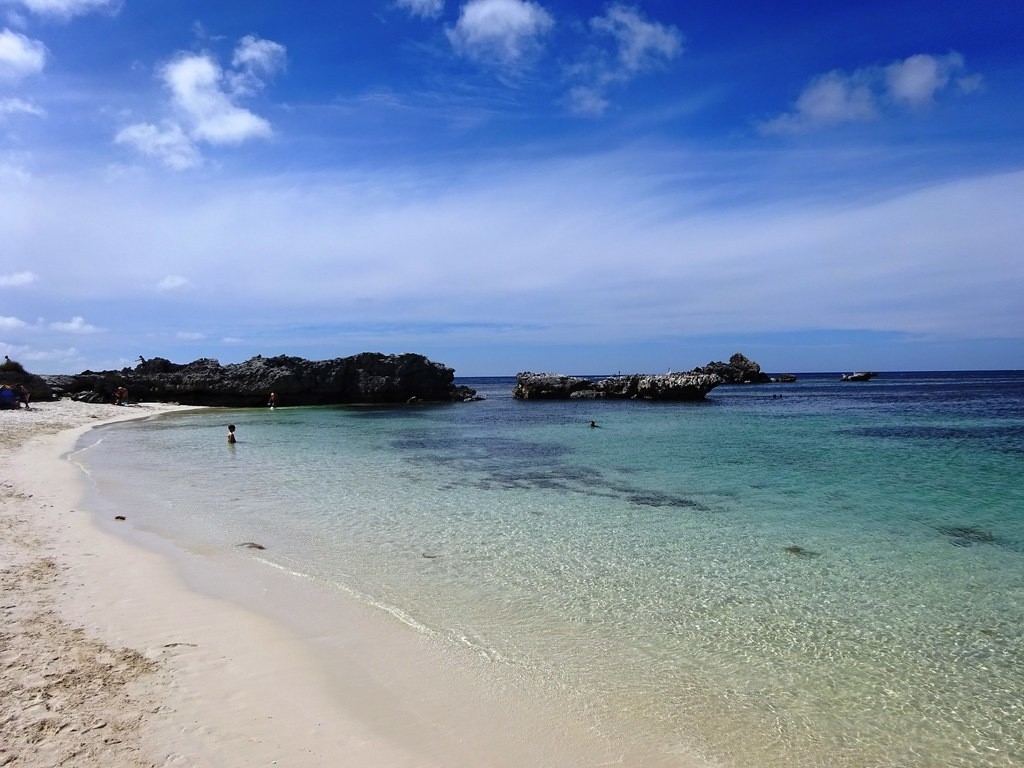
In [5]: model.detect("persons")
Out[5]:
[227,425,236,444]
[5,356,10,362]
[589,420,596,427]
[135,355,145,364]
[268,391,276,408]
[11,383,30,409]
[115,386,129,404]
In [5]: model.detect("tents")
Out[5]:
[0,384,16,410]
